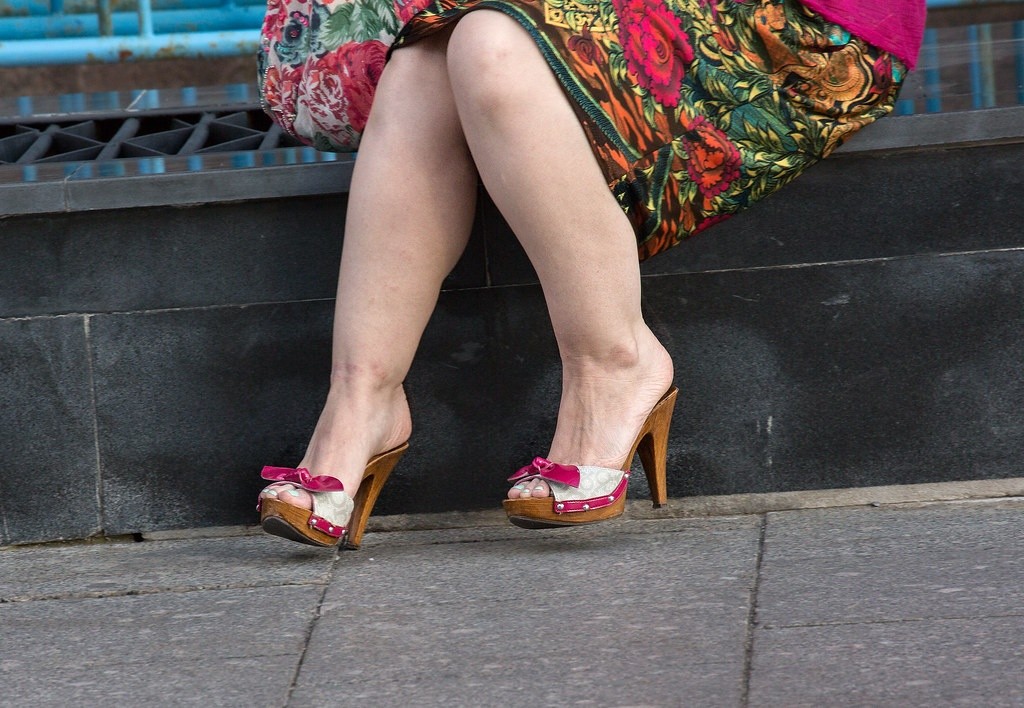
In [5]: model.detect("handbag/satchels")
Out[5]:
[257,0,434,153]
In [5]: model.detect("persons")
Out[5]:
[254,0,929,550]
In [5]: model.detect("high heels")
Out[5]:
[256,441,409,550]
[502,386,679,529]
